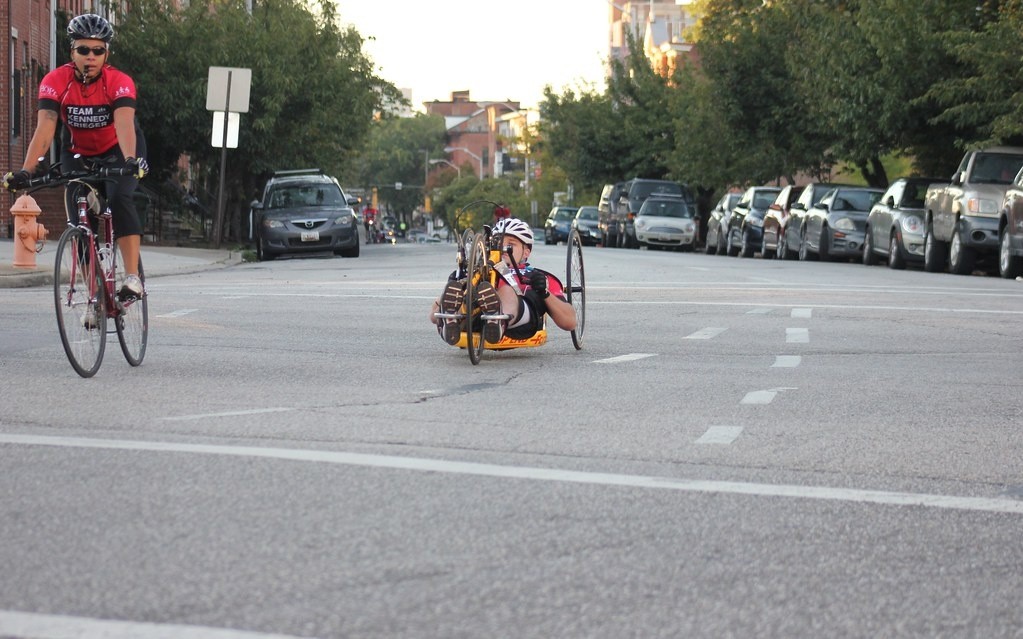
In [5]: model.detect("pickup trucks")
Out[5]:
[923,146,1023,274]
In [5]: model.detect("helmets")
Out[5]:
[67,14,113,43]
[492,218,535,244]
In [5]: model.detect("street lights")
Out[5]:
[470,101,531,196]
[443,144,484,182]
[429,157,461,180]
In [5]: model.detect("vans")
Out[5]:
[598,178,694,249]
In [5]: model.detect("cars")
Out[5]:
[799,187,886,261]
[864,177,952,269]
[543,206,602,245]
[631,194,702,252]
[378,215,441,243]
[250,168,361,260]
[999,164,1023,279]
[705,183,864,260]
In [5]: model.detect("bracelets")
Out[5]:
[435,301,441,306]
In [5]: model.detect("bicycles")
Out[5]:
[12,153,150,378]
[364,218,377,244]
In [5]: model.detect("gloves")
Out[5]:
[3,172,28,193]
[448,267,467,286]
[131,157,149,181]
[530,270,550,300]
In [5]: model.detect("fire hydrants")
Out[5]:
[10,191,50,267]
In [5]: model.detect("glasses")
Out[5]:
[73,45,107,56]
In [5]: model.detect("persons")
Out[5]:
[430,219,577,346]
[4,13,149,331]
[492,202,511,223]
[362,203,378,244]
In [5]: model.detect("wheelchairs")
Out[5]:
[433,200,587,365]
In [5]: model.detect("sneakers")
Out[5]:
[476,282,503,344]
[120,275,143,300]
[80,309,97,329]
[442,280,464,345]
